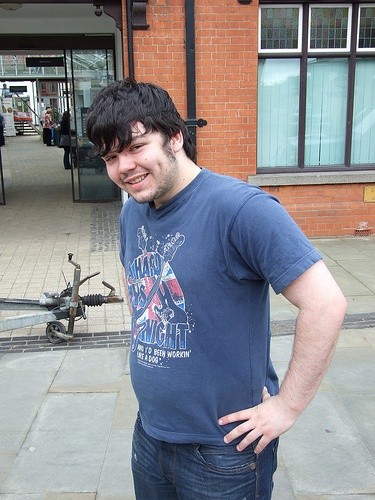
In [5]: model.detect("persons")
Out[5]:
[39,105,53,147]
[58,110,74,170]
[83,79,348,500]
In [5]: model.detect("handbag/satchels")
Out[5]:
[60,135,70,147]
